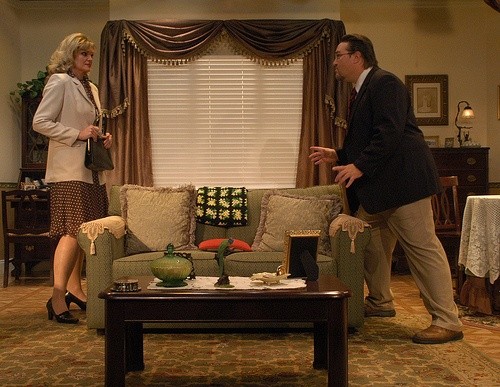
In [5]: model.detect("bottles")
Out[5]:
[150,243,192,287]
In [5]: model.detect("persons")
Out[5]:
[308,33,463,344]
[32,32,113,324]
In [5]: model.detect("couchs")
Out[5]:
[77,184,371,332]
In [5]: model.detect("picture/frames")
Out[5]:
[424,136,439,148]
[445,138,454,148]
[405,74,449,126]
[282,229,322,278]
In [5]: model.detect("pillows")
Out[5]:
[120,184,198,256]
[199,239,252,252]
[251,190,343,254]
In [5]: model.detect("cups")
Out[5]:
[445,137,454,147]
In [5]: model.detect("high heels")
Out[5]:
[64,290,86,312]
[47,297,79,324]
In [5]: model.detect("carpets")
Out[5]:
[0,312,500,387]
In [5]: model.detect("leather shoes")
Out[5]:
[364,301,396,317]
[413,324,463,344]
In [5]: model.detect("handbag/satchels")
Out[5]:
[85,112,114,171]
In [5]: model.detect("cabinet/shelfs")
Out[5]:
[10,72,51,276]
[430,148,490,274]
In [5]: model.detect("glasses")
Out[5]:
[334,53,357,60]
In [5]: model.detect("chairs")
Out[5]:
[432,177,464,294]
[1,190,50,287]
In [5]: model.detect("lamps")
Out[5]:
[455,101,474,147]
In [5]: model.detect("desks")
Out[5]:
[458,195,500,314]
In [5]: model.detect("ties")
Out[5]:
[349,88,357,124]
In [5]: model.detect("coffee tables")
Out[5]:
[98,276,353,386]
